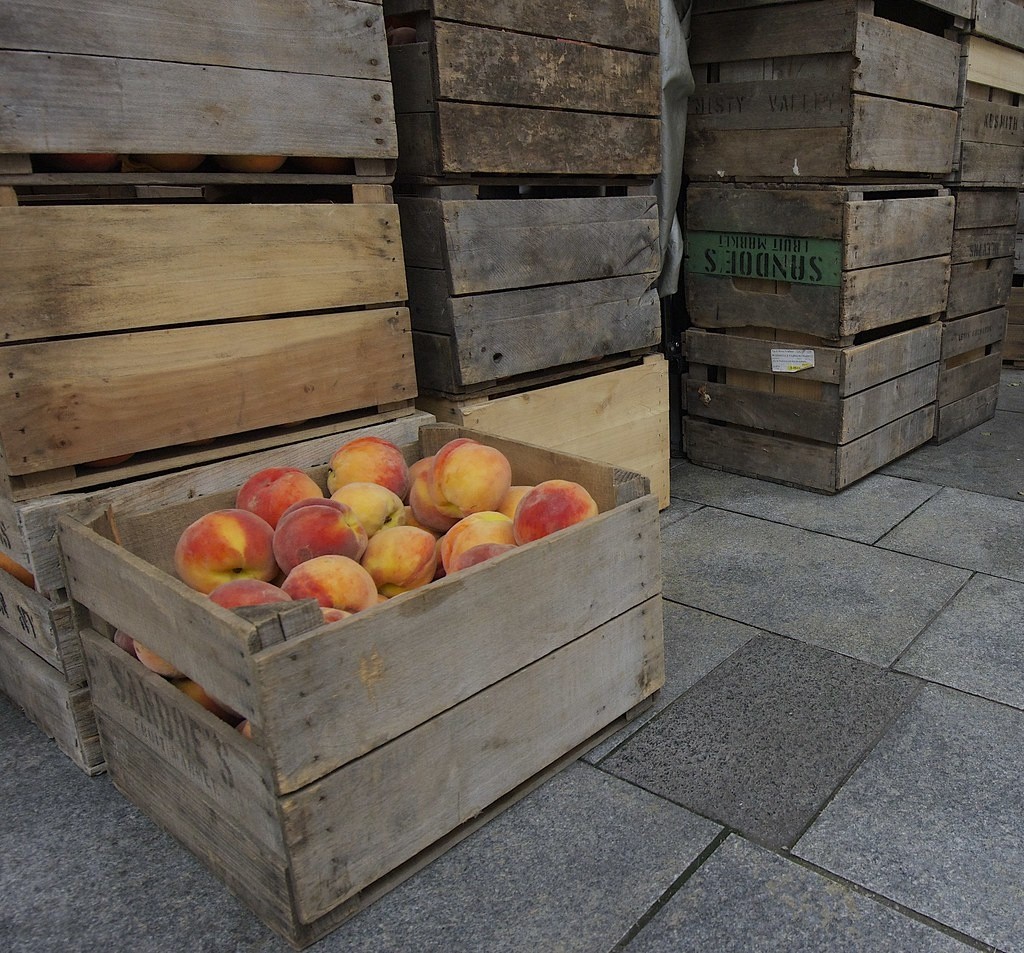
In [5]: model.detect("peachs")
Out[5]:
[110,437,601,737]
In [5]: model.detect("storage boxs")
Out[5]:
[0,0,1024,952]
